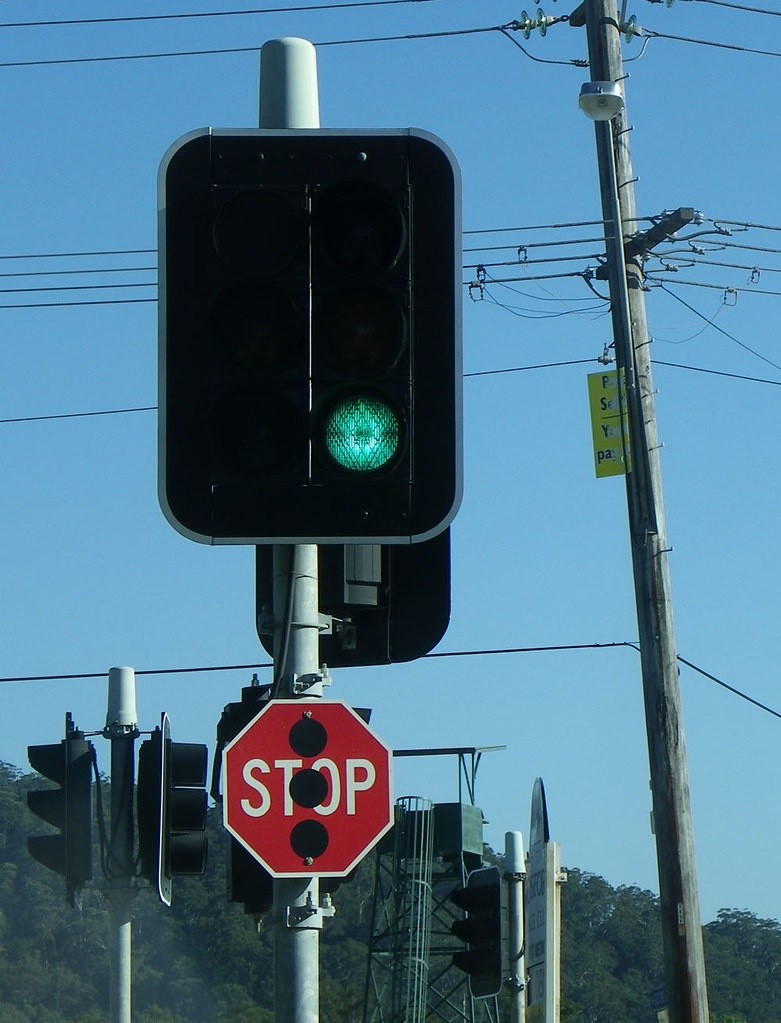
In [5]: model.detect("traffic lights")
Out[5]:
[451,866,504,999]
[26,710,91,884]
[137,710,207,910]
[154,130,465,547]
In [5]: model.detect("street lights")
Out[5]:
[575,77,712,1023]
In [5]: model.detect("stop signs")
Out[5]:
[221,698,396,879]
